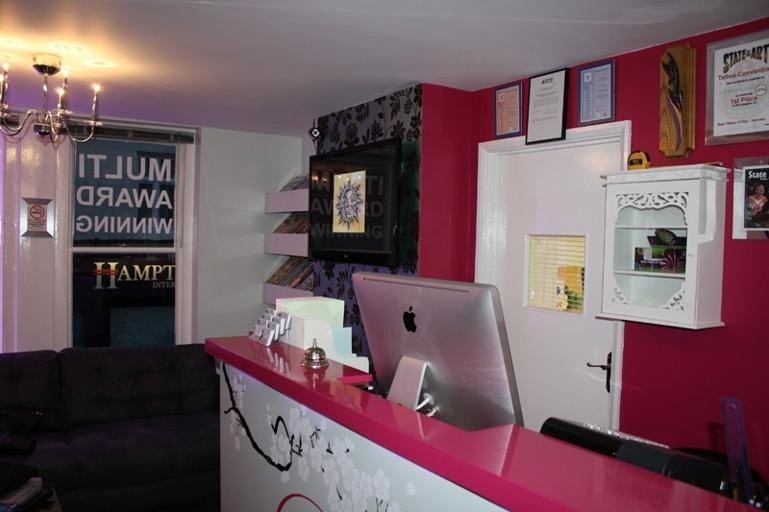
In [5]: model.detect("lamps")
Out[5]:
[0,52,103,146]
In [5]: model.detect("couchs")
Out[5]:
[59,344,220,511]
[0,349,79,512]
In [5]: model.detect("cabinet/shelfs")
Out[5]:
[595,163,732,330]
[263,188,313,306]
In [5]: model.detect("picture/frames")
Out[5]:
[525,68,568,145]
[495,82,522,138]
[577,58,615,127]
[704,28,768,147]
[731,155,769,241]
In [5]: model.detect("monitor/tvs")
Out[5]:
[308,136,402,268]
[351,272,524,432]
